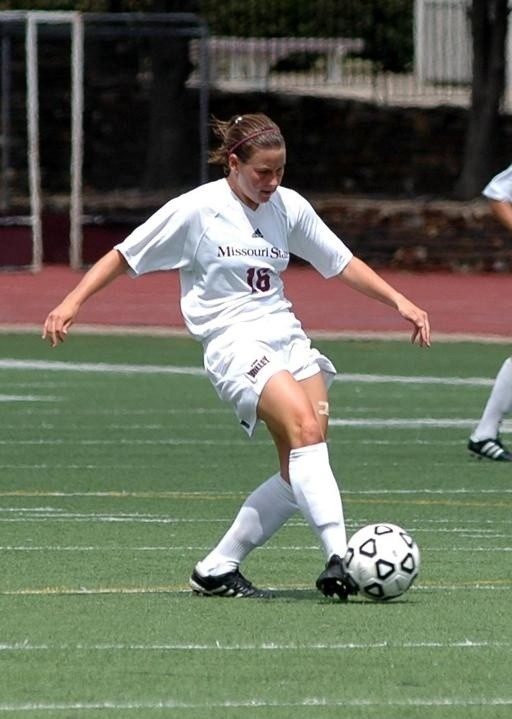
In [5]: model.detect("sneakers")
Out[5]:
[467,436,511,462]
[316,555,360,601]
[188,561,275,600]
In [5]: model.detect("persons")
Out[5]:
[464,165,512,464]
[36,112,433,604]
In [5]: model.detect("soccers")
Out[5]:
[341,522,422,600]
